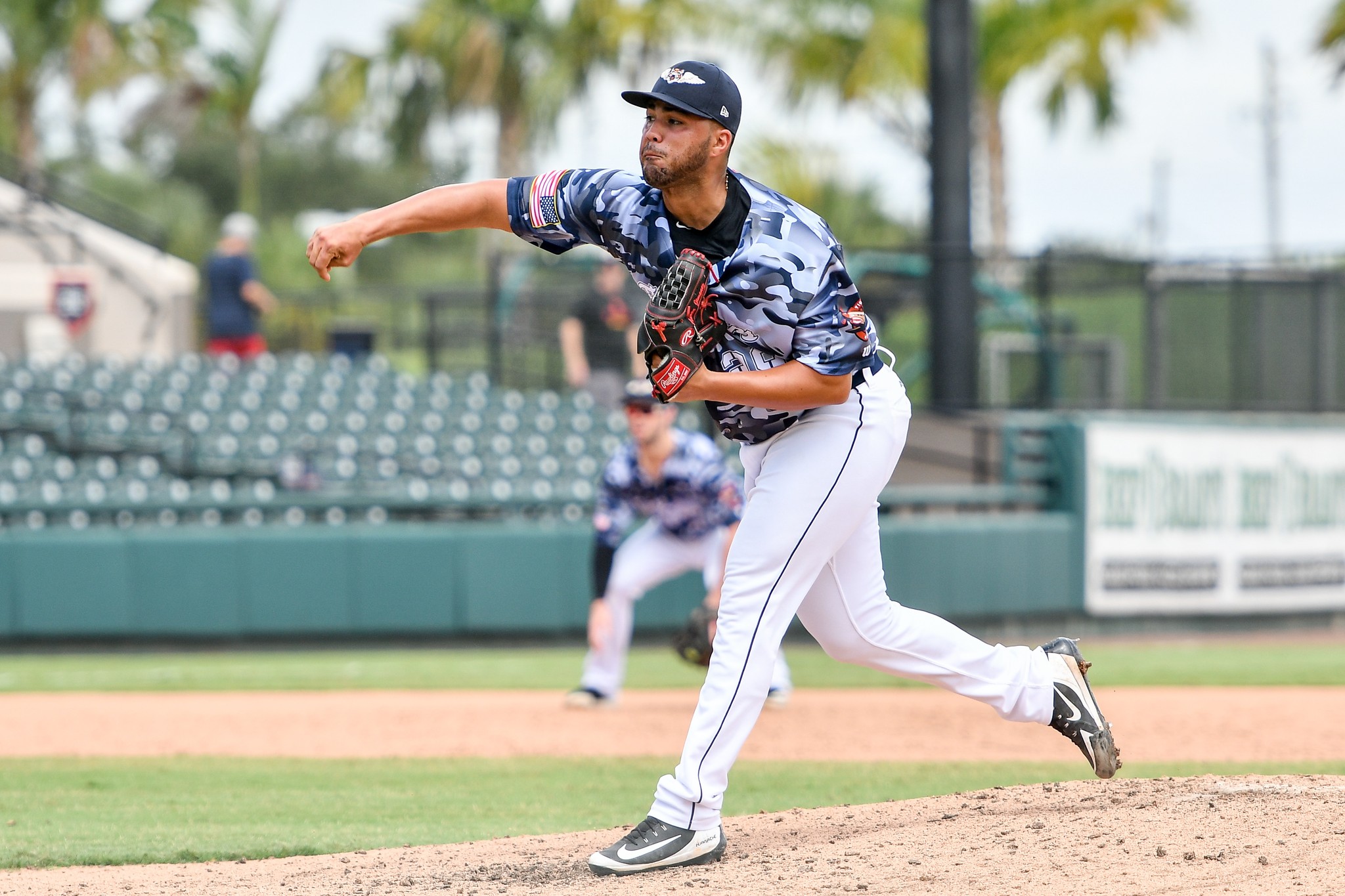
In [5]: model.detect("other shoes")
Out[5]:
[767,684,792,698]
[570,688,616,703]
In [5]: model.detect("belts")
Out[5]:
[801,350,883,414]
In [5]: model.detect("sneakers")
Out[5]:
[588,816,727,876]
[1042,635,1122,779]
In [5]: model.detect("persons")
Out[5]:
[568,376,793,713]
[301,59,1121,877]
[554,252,645,405]
[195,210,281,355]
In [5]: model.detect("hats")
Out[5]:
[621,60,742,137]
[620,379,663,405]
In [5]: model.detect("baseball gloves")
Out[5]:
[665,600,718,667]
[634,247,730,406]
